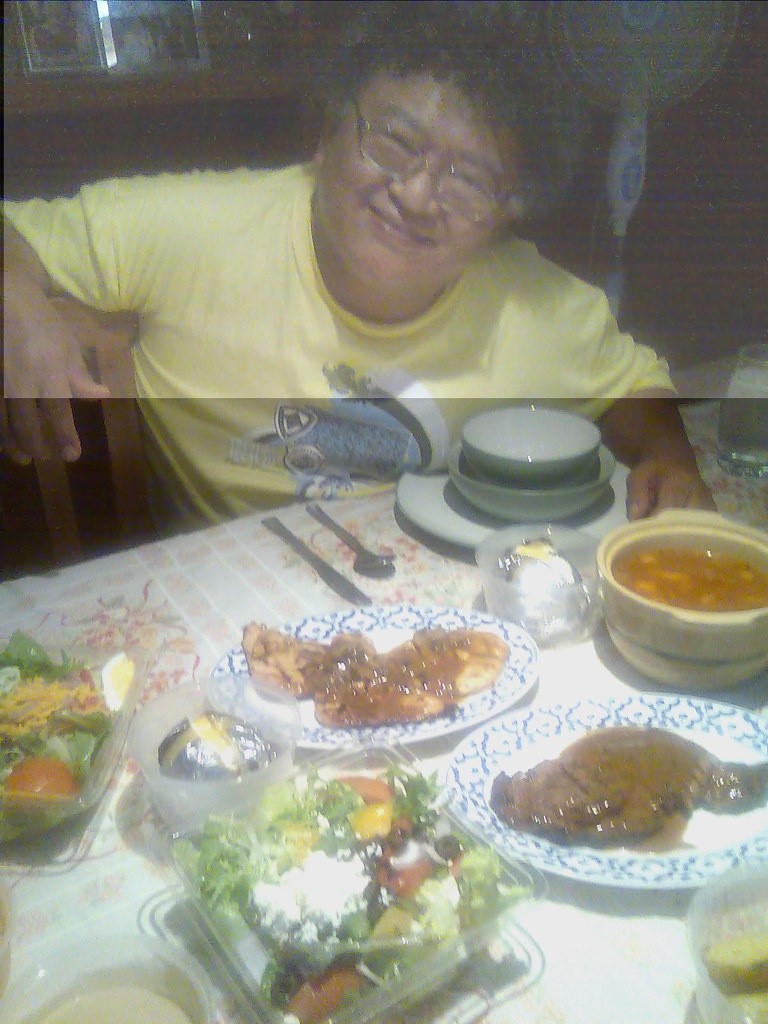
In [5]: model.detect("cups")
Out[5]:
[688,859,768,1024]
[473,521,602,645]
[123,674,303,832]
[715,343,768,478]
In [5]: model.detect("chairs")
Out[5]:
[34,296,160,567]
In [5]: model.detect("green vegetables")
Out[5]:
[176,756,524,1023]
[0,631,121,844]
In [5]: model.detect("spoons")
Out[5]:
[315,491,396,580]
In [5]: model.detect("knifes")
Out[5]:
[263,515,372,607]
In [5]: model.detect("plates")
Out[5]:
[195,609,544,752]
[397,466,636,559]
[441,688,768,889]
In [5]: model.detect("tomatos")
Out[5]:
[284,969,363,1022]
[337,778,395,811]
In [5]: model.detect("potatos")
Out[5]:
[697,936,767,1024]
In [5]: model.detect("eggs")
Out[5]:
[100,651,135,712]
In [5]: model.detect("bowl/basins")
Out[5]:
[462,406,603,489]
[596,507,768,688]
[445,436,614,522]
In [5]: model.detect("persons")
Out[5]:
[0,25,717,540]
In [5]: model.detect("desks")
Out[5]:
[2,382,767,1021]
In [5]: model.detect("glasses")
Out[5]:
[341,76,509,225]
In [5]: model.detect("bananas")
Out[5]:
[5,758,82,801]
